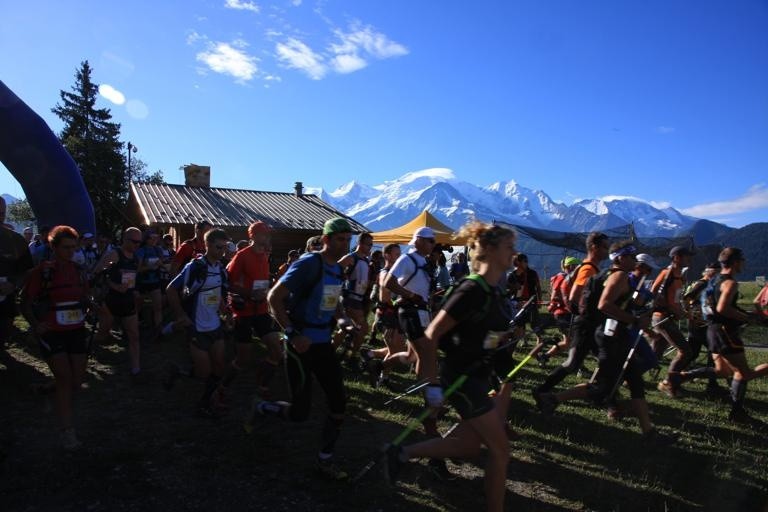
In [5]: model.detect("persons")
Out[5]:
[2,196,767,512]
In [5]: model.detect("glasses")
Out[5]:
[216,243,225,249]
[424,237,435,243]
[126,239,141,244]
[737,257,746,261]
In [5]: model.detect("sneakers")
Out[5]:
[537,351,550,370]
[668,371,682,397]
[211,383,231,411]
[656,379,684,398]
[603,393,626,420]
[55,424,82,452]
[641,427,681,447]
[383,442,402,490]
[426,459,458,482]
[535,390,561,427]
[532,379,553,400]
[28,383,42,401]
[367,358,383,389]
[130,368,148,384]
[231,358,241,369]
[311,452,348,480]
[728,407,756,424]
[577,368,593,378]
[239,393,264,433]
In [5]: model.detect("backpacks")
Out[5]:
[577,267,624,323]
[677,276,716,327]
[544,261,596,316]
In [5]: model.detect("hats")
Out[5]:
[414,226,436,238]
[565,256,581,267]
[322,217,358,235]
[248,222,274,239]
[635,253,661,270]
[669,245,697,257]
